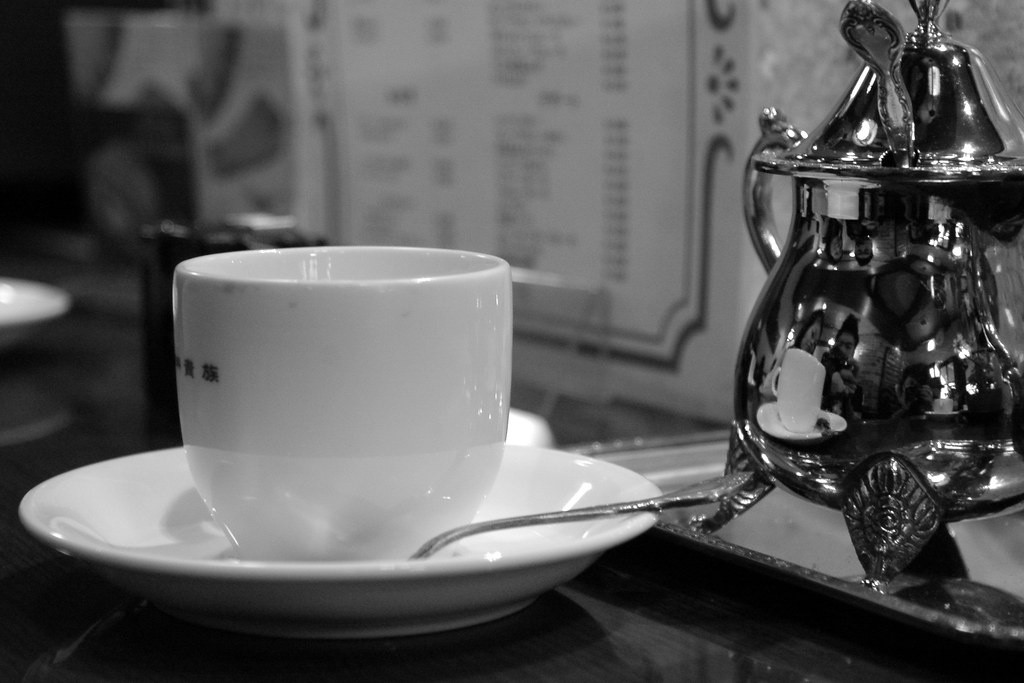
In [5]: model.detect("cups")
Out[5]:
[170,243,512,565]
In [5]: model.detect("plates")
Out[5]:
[0,275,74,347]
[17,445,665,637]
